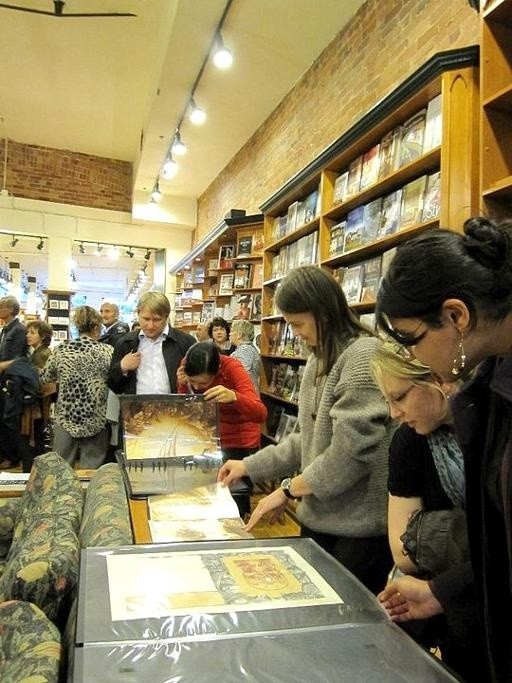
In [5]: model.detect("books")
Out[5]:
[147,480,255,544]
[74,537,461,682]
[118,393,249,500]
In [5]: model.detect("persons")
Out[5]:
[176,341,267,518]
[0,297,141,503]
[217,266,393,597]
[228,320,265,392]
[373,215,511,683]
[208,316,237,357]
[369,332,490,682]
[195,322,213,345]
[107,291,195,462]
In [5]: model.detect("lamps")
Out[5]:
[73,236,159,262]
[140,0,232,212]
[0,231,49,250]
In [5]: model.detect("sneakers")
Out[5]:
[0,460,13,469]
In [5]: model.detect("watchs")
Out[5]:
[281,477,302,502]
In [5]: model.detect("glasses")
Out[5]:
[393,322,428,357]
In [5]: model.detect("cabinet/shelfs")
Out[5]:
[479,0,512,225]
[40,298,74,343]
[264,45,478,446]
[172,214,264,351]
